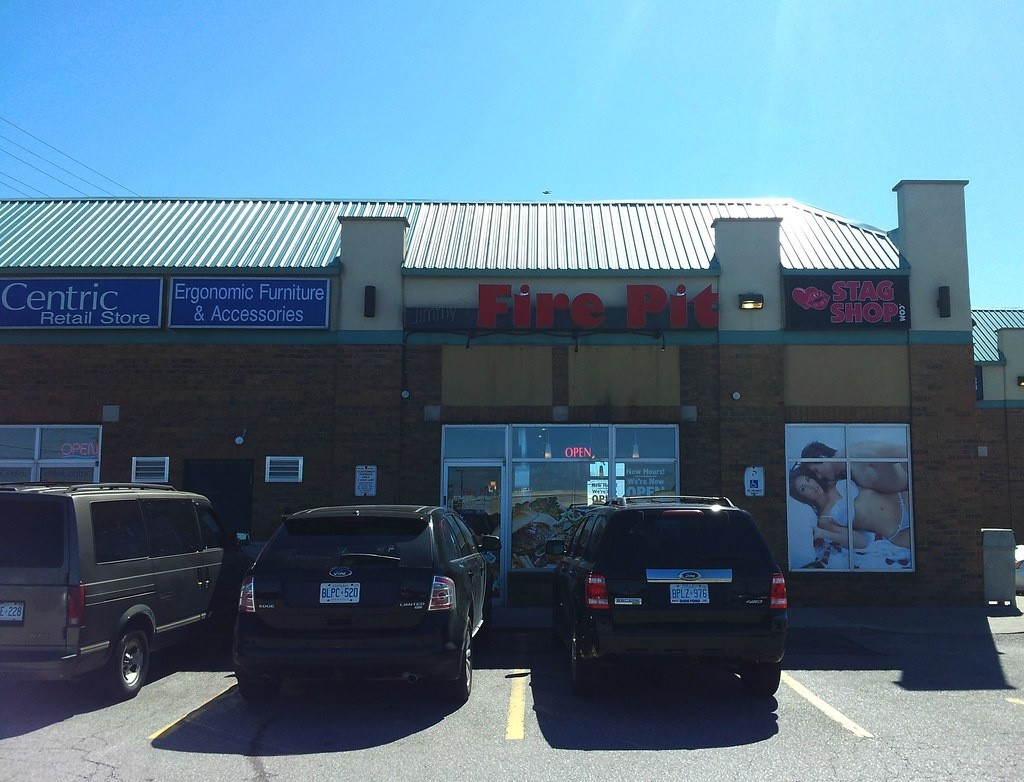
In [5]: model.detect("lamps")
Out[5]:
[738,288,765,310]
[542,430,555,462]
[630,430,640,459]
[1015,375,1024,387]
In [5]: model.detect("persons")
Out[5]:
[799,439,909,494]
[788,467,912,551]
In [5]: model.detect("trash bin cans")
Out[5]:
[981,527,1017,607]
